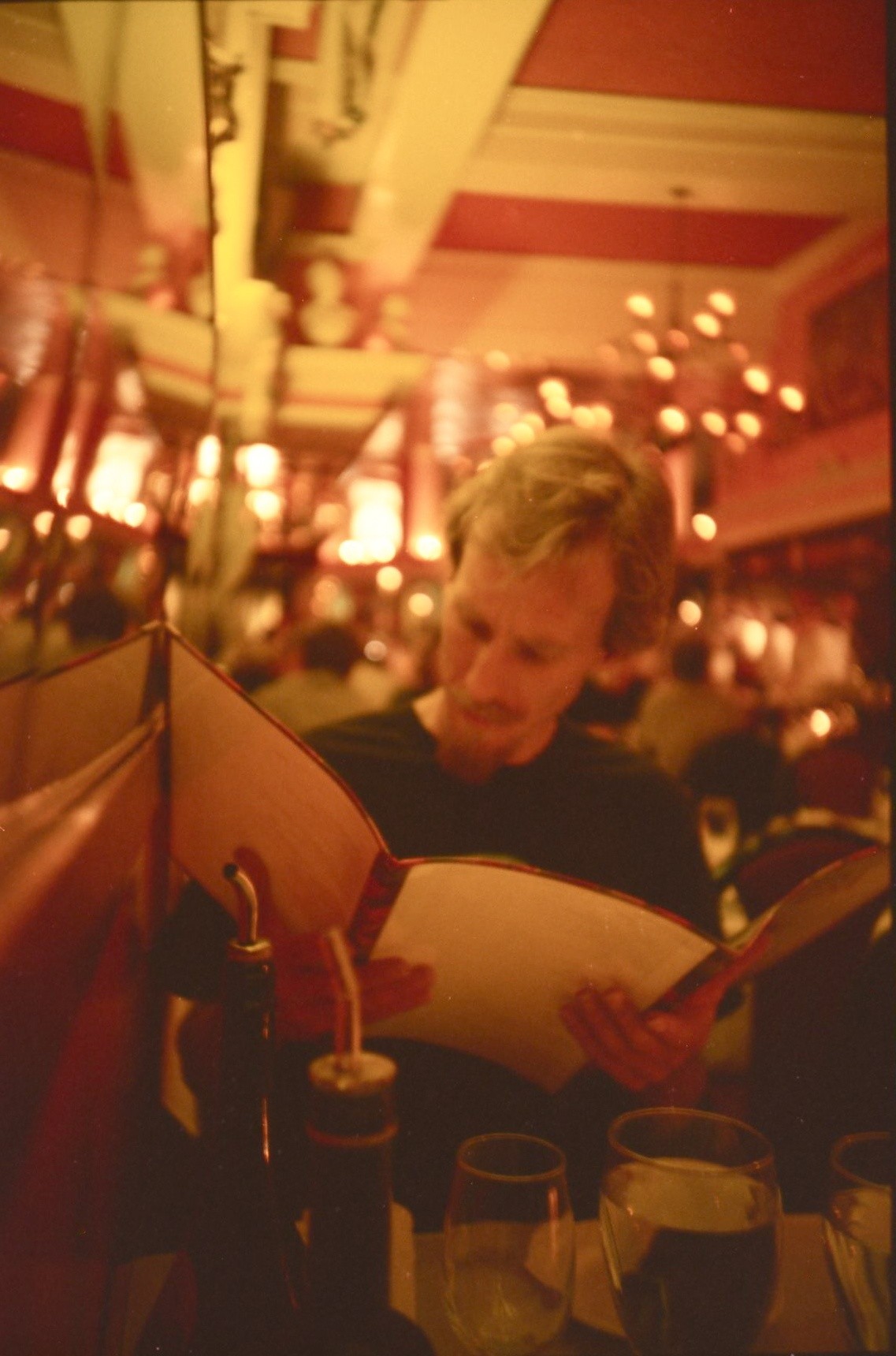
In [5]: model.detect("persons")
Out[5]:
[239,629,359,744]
[282,427,770,1154]
[39,584,129,675]
[572,631,896,849]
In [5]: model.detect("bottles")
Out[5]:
[150,862,435,1355]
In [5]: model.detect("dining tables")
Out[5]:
[122,1203,869,1356]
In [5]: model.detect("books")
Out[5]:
[0,622,886,1108]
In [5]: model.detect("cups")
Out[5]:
[441,1091,896,1356]
